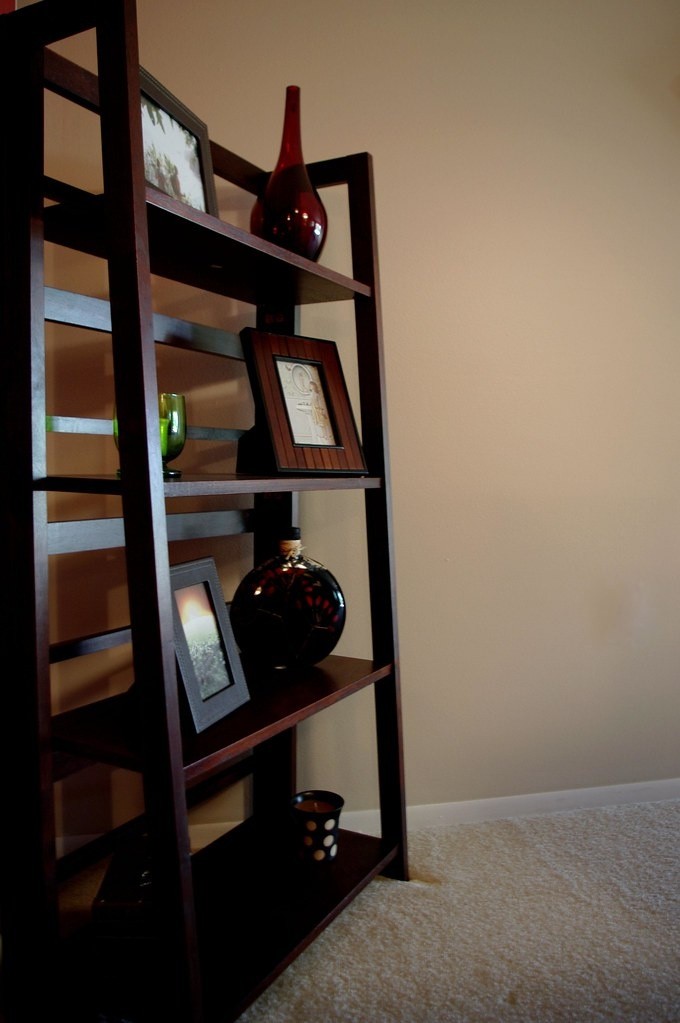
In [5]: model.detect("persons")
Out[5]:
[153,157,186,201]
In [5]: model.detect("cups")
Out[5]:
[288,789,345,865]
[113,393,188,478]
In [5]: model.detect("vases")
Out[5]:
[248,84,329,261]
[227,527,346,673]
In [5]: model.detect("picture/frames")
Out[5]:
[240,325,370,477]
[139,66,217,218]
[168,559,250,735]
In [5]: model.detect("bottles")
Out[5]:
[250,86,328,262]
[230,527,346,672]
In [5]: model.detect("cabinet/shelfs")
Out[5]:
[0,0,410,1023]
